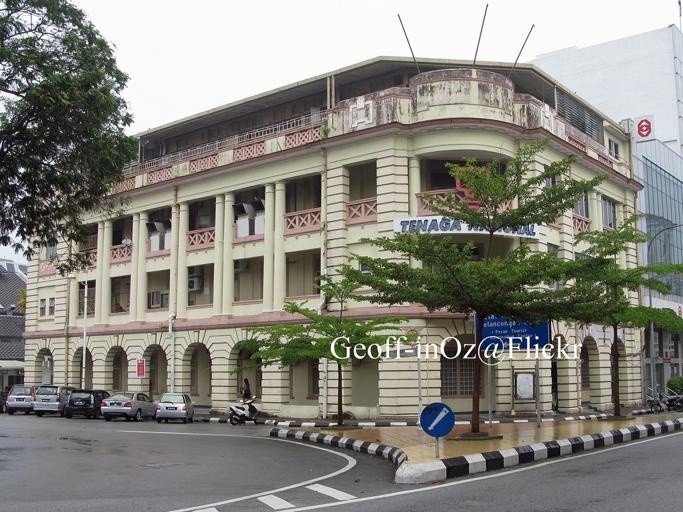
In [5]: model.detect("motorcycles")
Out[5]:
[229,395,260,426]
[649,392,683,414]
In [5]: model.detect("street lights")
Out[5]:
[64,272,76,388]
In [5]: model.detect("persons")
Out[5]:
[115,303,124,312]
[242,378,251,405]
[121,235,131,255]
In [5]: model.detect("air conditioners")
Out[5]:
[234,259,249,272]
[188,278,202,292]
[188,265,201,277]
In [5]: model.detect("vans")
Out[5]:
[0,383,111,419]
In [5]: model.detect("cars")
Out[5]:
[155,391,195,423]
[100,390,159,422]
